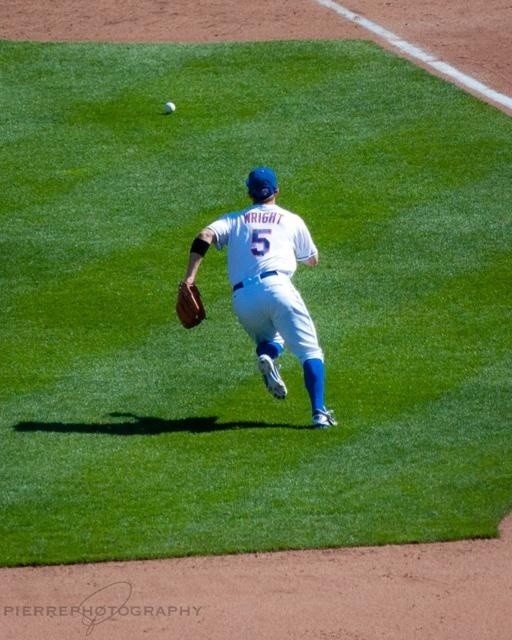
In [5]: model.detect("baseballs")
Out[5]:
[165,102,175,113]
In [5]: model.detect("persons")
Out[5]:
[176,164,340,429]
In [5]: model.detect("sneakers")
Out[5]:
[258,353,287,400]
[312,409,337,429]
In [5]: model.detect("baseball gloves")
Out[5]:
[177,280,206,329]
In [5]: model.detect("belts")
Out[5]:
[233,271,277,292]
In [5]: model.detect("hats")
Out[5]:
[246,167,278,197]
